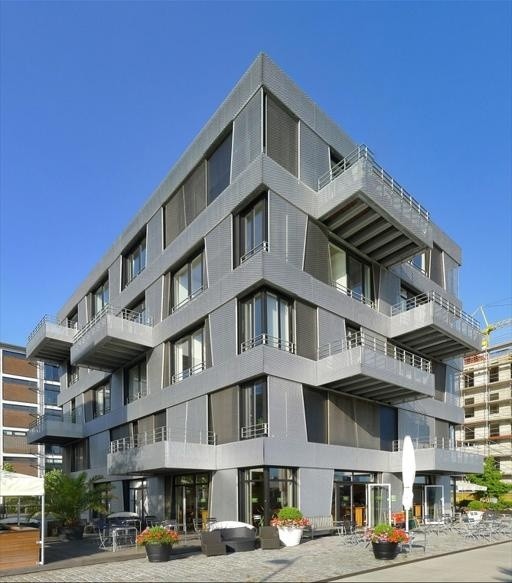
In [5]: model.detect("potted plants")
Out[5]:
[465,499,487,525]
[44,468,119,539]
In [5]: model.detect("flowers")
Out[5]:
[135,525,180,546]
[270,509,312,528]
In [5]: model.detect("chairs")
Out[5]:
[258,526,281,550]
[200,530,226,557]
[334,513,512,554]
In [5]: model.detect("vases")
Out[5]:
[272,524,304,546]
[370,542,398,560]
[145,543,173,561]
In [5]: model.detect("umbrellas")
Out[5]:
[402,434,416,542]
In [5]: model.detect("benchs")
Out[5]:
[216,527,257,552]
[305,514,346,540]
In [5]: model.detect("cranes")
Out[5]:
[476,304,511,352]
